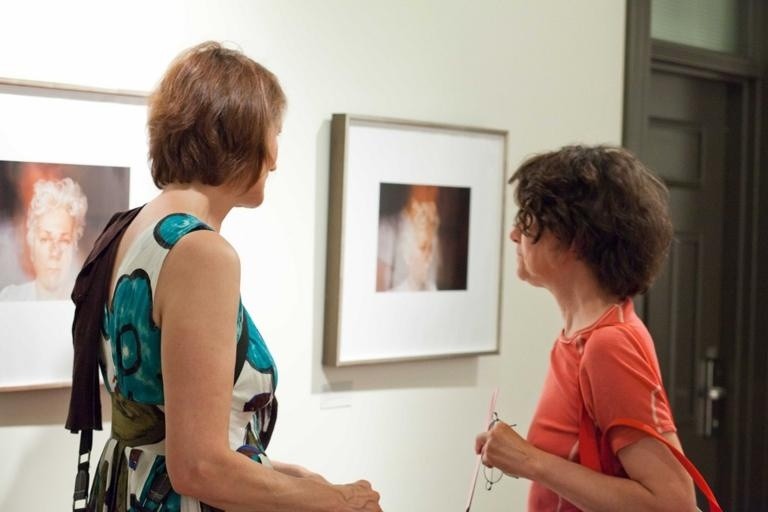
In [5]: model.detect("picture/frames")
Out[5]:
[1,79,148,393]
[321,113,510,365]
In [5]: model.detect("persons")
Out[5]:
[0,177,95,305]
[87,39,382,511]
[378,182,444,295]
[0,160,63,293]
[472,139,699,511]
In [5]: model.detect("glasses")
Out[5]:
[483,411,519,493]
